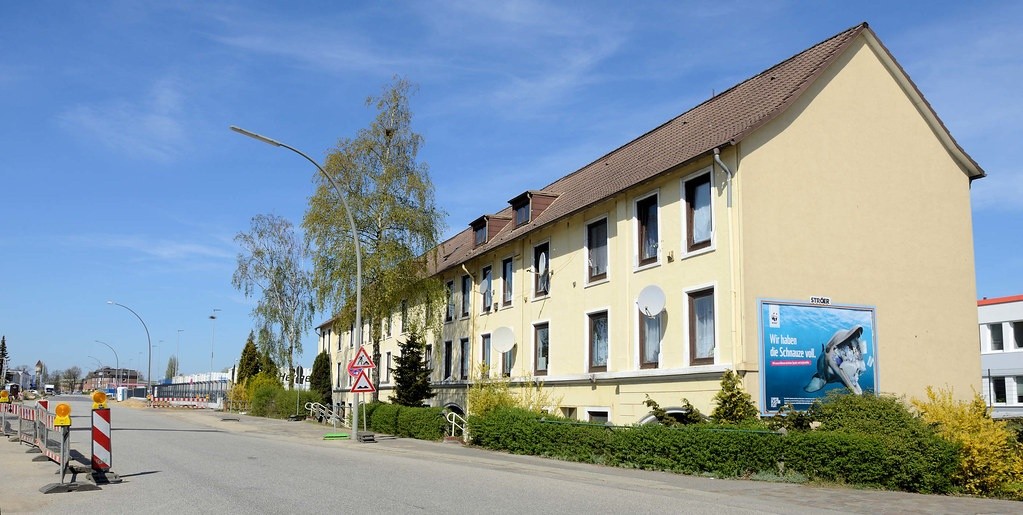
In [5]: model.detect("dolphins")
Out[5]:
[804,326,864,395]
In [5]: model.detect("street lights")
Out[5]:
[175,329,185,377]
[87,355,104,387]
[127,358,134,389]
[136,352,143,386]
[95,341,118,388]
[107,301,152,391]
[210,308,222,381]
[157,340,164,379]
[230,124,364,440]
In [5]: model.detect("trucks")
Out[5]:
[44,384,55,396]
